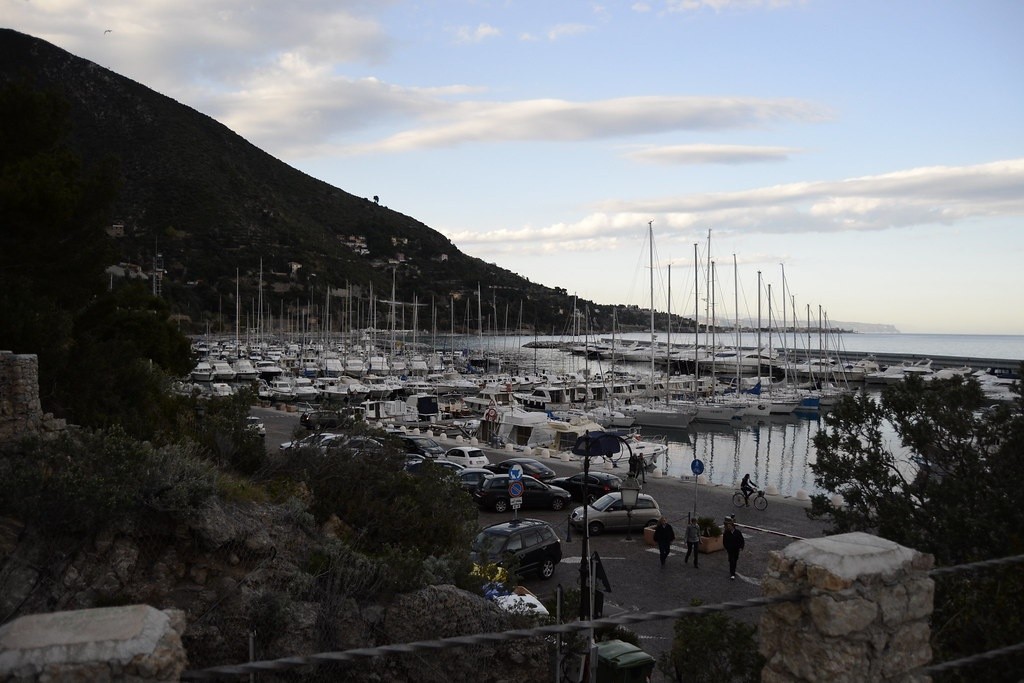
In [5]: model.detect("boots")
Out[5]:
[661,559,665,569]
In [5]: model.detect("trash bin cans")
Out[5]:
[594,638,655,683]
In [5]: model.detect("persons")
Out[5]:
[636,453,647,484]
[723,521,744,579]
[629,454,638,478]
[653,517,675,565]
[741,474,759,507]
[684,518,703,568]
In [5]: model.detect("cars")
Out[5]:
[279,432,335,457]
[444,446,490,468]
[483,457,557,482]
[397,434,445,461]
[450,467,496,497]
[569,491,662,536]
[548,471,623,506]
[242,416,266,437]
[333,436,404,463]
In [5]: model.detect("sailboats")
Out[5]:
[188,218,1024,471]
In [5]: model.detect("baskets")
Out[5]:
[757,491,765,497]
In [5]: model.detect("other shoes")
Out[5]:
[694,566,698,568]
[685,557,688,563]
[731,576,735,579]
[643,481,647,483]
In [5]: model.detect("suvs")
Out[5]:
[468,518,563,581]
[474,474,572,514]
[300,409,354,431]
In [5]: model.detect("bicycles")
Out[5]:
[732,487,768,511]
